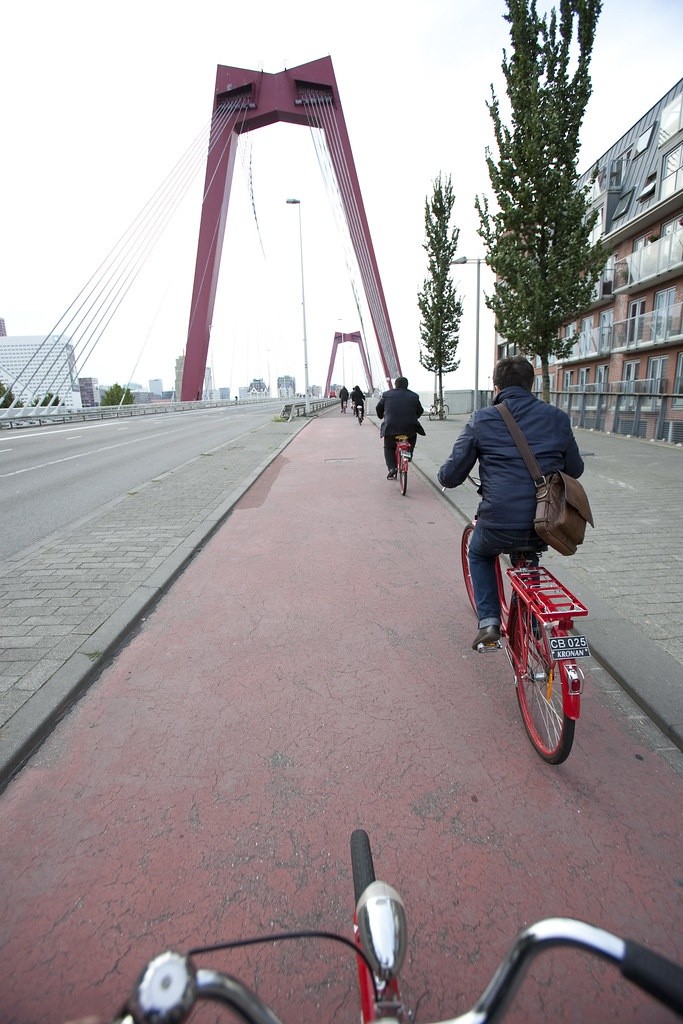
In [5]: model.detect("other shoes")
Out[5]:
[408,454,412,462]
[387,470,395,479]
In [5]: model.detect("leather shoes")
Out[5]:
[532,623,542,640]
[472,624,500,650]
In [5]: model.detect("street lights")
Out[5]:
[286,198,310,417]
[452,256,480,416]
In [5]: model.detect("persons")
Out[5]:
[339,386,349,411]
[375,377,426,480]
[437,356,584,650]
[350,385,365,420]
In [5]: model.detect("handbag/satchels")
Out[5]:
[534,470,594,556]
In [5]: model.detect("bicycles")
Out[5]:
[441,471,592,765]
[352,400,356,415]
[356,405,363,425]
[342,399,347,414]
[381,413,412,496]
[107,828,683,1024]
[429,397,449,421]
